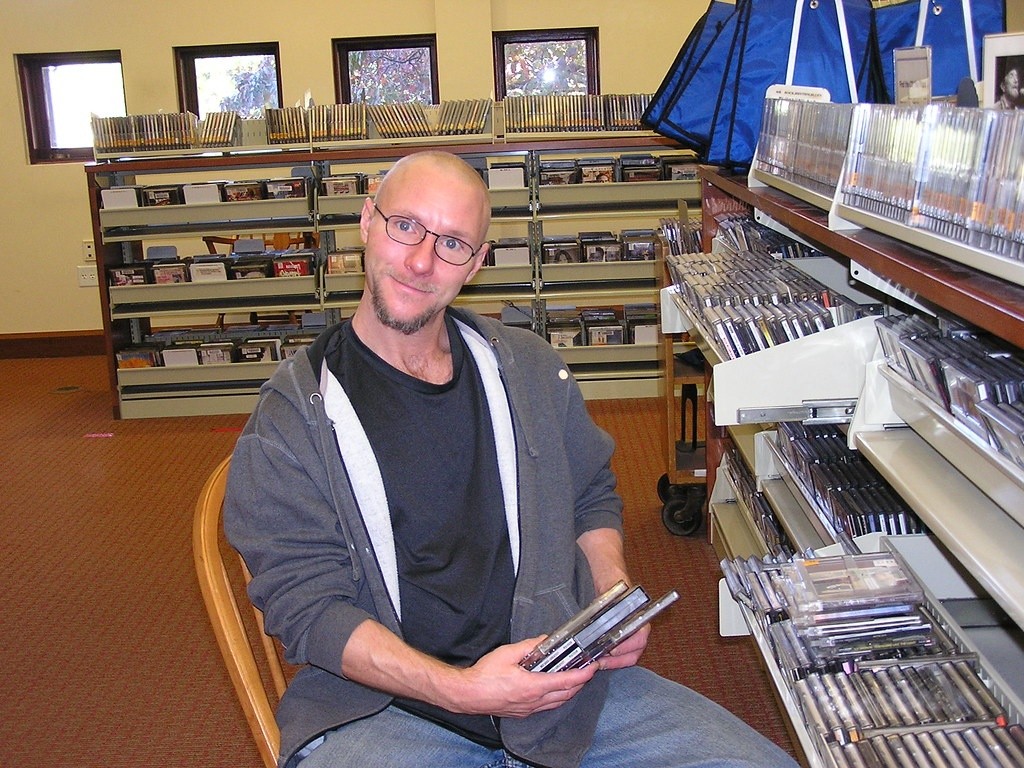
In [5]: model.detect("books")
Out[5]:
[266,104,366,145]
[434,98,492,136]
[368,102,434,139]
[90,110,237,152]
[505,93,660,133]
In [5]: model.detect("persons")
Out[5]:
[554,250,573,263]
[995,68,1024,108]
[224,150,801,768]
[596,173,610,183]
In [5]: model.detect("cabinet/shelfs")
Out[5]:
[84,33,1024,768]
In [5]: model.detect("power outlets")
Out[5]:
[83,240,96,262]
[77,265,99,287]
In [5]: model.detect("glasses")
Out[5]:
[374,204,482,266]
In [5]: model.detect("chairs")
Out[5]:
[192,452,307,768]
[201,233,313,327]
